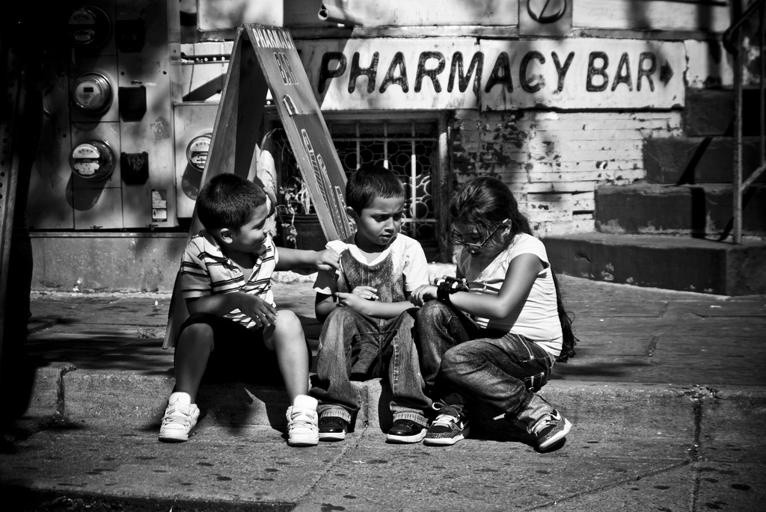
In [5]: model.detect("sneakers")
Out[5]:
[423,404,470,445]
[317,417,345,439]
[286,394,319,445]
[530,409,572,448]
[387,420,426,443]
[159,391,200,441]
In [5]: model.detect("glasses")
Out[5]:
[448,225,500,250]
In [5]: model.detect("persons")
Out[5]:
[159,172,344,444]
[409,175,579,451]
[313,161,431,443]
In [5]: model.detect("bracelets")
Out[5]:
[438,274,470,307]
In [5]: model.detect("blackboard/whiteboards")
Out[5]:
[244,22,360,243]
